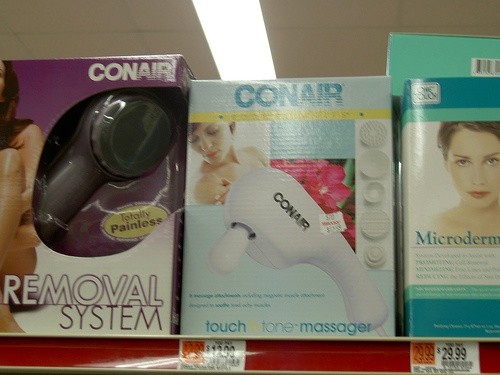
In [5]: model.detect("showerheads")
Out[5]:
[34,92,178,242]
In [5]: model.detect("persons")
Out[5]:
[436,119,500,247]
[1,59,45,334]
[188,119,270,206]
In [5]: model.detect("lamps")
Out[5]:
[187,0,277,82]
